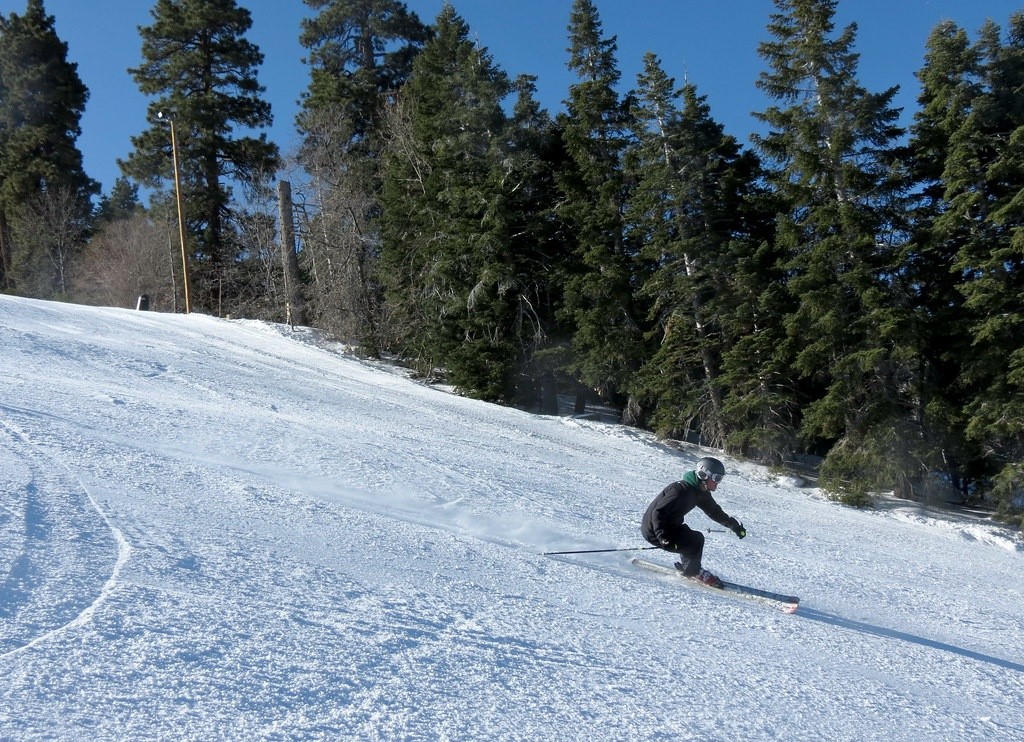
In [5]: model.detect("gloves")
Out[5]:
[736,522,746,539]
[670,544,678,549]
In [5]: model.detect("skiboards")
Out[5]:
[628,557,800,618]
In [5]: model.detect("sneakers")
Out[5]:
[690,567,715,584]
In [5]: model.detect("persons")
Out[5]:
[640,457,747,590]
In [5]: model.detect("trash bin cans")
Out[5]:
[136,295,150,312]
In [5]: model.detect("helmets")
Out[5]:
[695,457,725,492]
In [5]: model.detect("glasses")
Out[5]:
[711,473,724,483]
